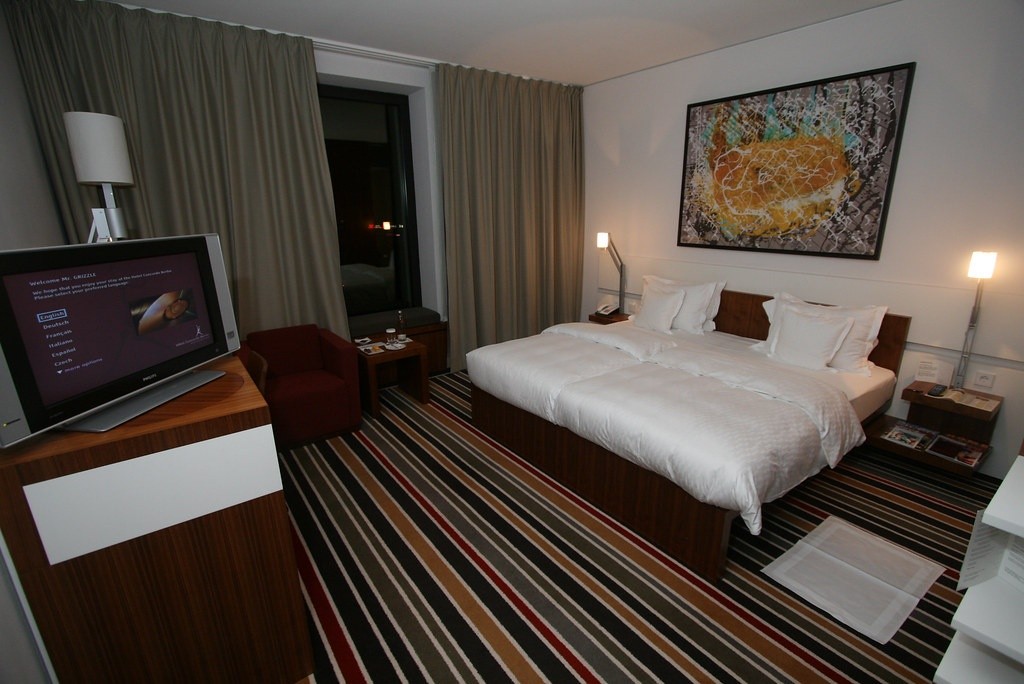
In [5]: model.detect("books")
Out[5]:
[923,384,1001,413]
[880,418,941,453]
[924,431,994,471]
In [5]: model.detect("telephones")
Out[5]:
[595,303,619,317]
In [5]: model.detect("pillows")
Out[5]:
[629,276,727,336]
[749,290,889,378]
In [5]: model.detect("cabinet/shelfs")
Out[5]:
[0,353,315,684]
[934,456,1024,684]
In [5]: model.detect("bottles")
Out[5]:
[398,311,406,334]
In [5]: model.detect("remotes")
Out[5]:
[928,384,947,396]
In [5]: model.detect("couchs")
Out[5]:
[232,323,362,452]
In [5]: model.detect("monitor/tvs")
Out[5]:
[0,234,241,450]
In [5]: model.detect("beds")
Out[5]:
[466,291,993,589]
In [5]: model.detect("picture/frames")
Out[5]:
[676,61,917,262]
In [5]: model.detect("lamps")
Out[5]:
[597,232,625,313]
[954,251,997,389]
[62,112,135,241]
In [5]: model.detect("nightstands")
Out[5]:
[589,313,631,325]
[901,380,1003,445]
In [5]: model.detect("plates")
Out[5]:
[396,337,413,344]
[357,342,387,355]
[386,344,405,350]
[354,337,372,344]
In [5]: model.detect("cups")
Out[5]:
[398,334,406,341]
[386,329,396,344]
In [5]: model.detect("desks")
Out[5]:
[358,334,430,418]
